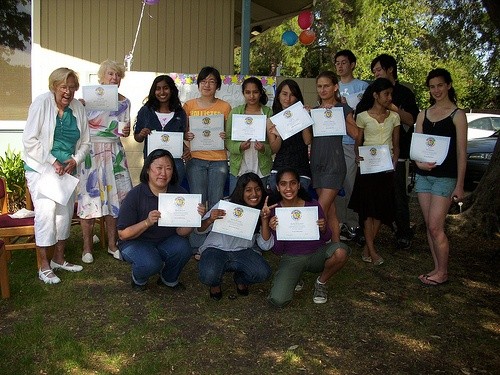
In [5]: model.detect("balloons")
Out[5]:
[299,30,315,46]
[297,8,314,30]
[281,30,298,46]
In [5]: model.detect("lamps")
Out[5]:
[250,26,262,35]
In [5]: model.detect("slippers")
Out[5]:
[422,273,449,286]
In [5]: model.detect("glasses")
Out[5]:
[56,85,76,93]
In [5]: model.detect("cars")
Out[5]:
[413,113,500,159]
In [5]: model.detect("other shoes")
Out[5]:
[361,256,372,262]
[82,252,93,263]
[50,260,84,273]
[39,267,60,284]
[236,280,249,296]
[397,237,411,248]
[373,255,384,265]
[158,278,185,290]
[131,277,146,291]
[108,247,120,259]
[209,285,222,300]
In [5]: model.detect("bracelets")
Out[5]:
[144,220,149,230]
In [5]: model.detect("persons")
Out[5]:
[352,77,401,267]
[267,79,312,201]
[133,75,192,193]
[354,54,420,250]
[115,148,206,293]
[182,66,232,261]
[334,49,370,241]
[309,70,358,243]
[22,67,91,283]
[412,67,468,286]
[194,171,277,300]
[224,77,275,196]
[78,59,133,263]
[264,167,351,306]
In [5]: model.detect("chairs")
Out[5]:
[0,176,105,298]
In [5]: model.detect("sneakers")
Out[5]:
[295,280,304,291]
[313,278,328,304]
